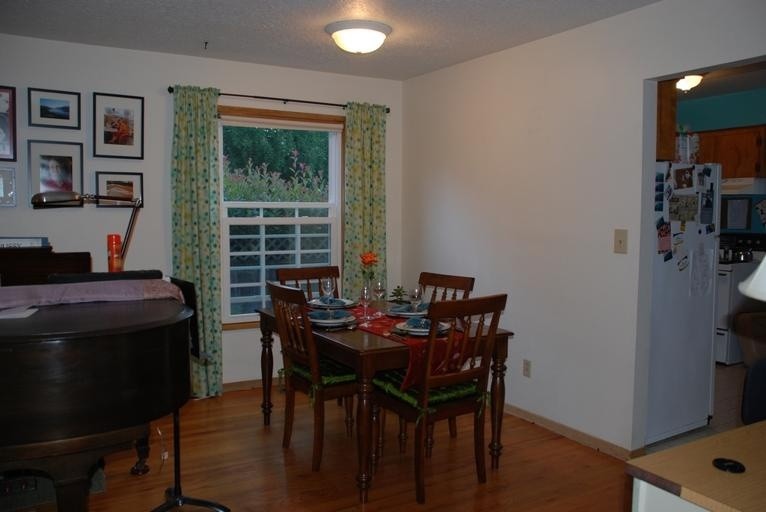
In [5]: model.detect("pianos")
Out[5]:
[0,297,195,512]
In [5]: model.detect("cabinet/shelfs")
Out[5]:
[688,124,766,183]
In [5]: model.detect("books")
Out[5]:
[0,303,37,320]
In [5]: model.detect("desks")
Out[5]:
[626,415,765,512]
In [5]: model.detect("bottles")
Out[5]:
[107,233,123,272]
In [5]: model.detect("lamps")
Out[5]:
[30,190,142,258]
[324,19,393,55]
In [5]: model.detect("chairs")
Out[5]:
[275,264,341,304]
[413,272,475,437]
[264,278,386,473]
[368,289,512,506]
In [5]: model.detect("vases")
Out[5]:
[364,275,372,301]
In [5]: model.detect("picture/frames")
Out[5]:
[0,168,16,207]
[94,169,144,209]
[26,85,82,131]
[93,91,146,159]
[0,85,17,162]
[25,137,85,210]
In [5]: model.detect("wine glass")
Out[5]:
[408,284,422,310]
[319,275,337,311]
[372,274,387,318]
[360,287,373,322]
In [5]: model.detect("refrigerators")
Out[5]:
[646,162,724,453]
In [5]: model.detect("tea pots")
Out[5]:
[720,244,755,263]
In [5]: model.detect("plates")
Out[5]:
[396,321,451,336]
[306,312,356,328]
[388,308,428,318]
[307,299,354,308]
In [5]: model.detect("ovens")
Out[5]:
[715,270,733,365]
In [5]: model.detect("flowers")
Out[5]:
[360,250,378,275]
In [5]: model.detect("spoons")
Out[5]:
[327,324,357,332]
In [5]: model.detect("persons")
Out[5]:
[41,160,71,191]
